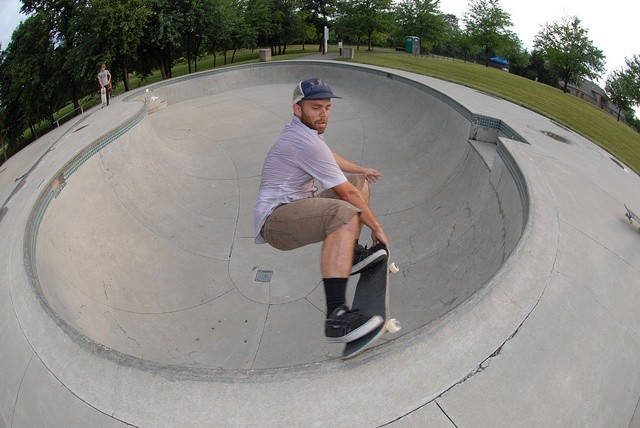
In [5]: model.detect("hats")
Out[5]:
[293,79,342,104]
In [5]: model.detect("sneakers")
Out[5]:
[325,305,383,342]
[349,240,388,276]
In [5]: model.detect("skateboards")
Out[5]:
[341,244,400,360]
[101,88,106,108]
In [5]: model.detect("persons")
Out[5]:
[254,77,389,343]
[97,62,112,106]
